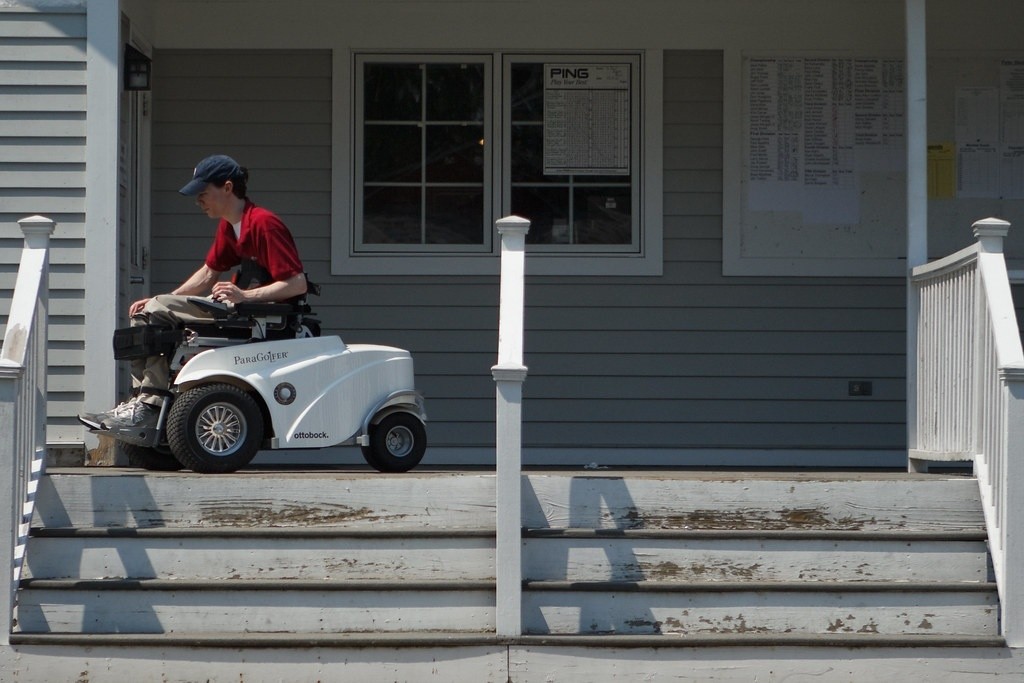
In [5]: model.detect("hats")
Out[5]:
[178,154,242,197]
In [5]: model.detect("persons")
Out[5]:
[76,152,309,447]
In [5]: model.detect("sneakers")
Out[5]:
[101,401,160,430]
[78,397,139,429]
